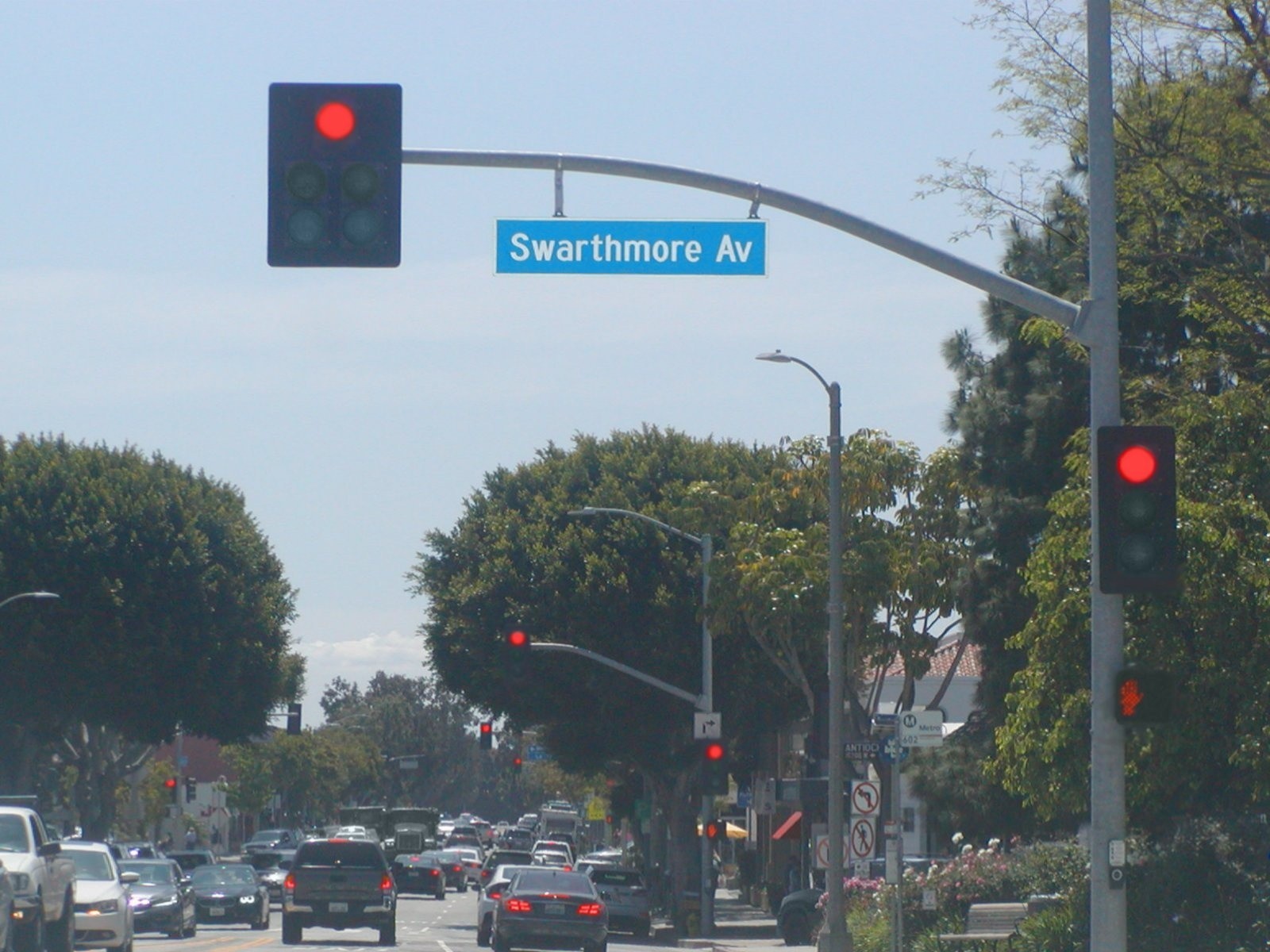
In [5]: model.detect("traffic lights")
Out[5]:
[480,723,493,751]
[702,741,728,793]
[1098,423,1179,590]
[1113,672,1175,720]
[709,824,719,837]
[166,775,176,800]
[514,756,521,772]
[271,79,403,270]
[508,628,530,650]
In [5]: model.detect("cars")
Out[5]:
[775,855,949,944]
[0,794,655,952]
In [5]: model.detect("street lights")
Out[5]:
[566,502,721,933]
[762,349,852,951]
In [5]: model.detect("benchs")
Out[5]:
[939,902,1029,952]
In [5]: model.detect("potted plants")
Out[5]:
[737,849,790,917]
